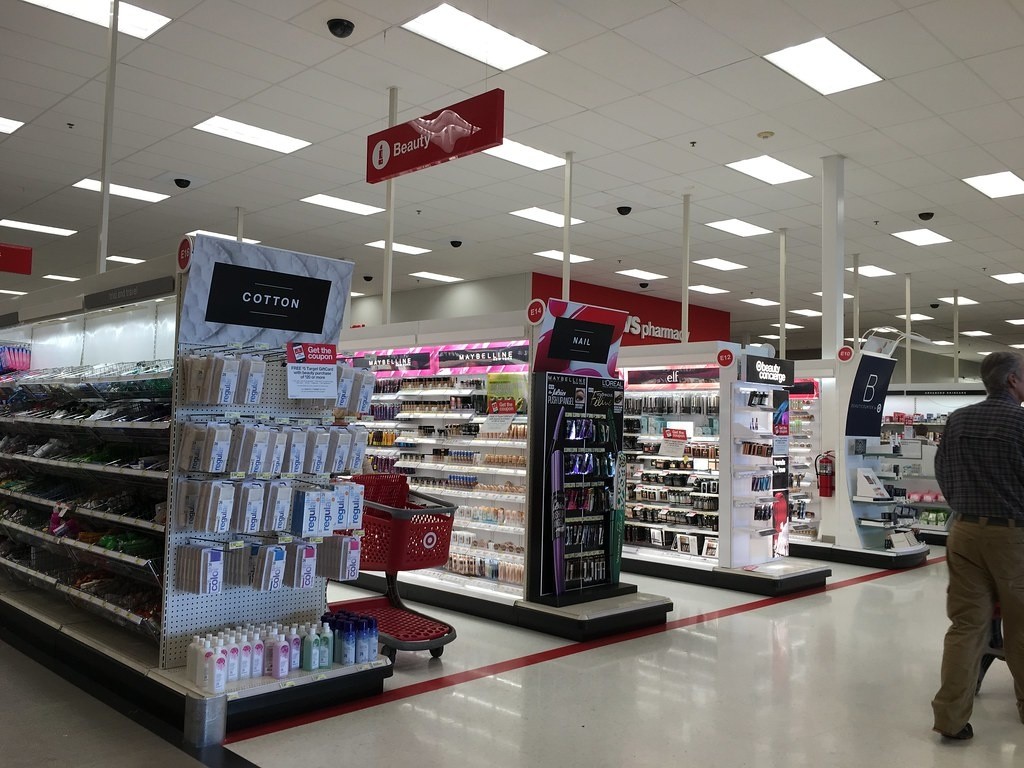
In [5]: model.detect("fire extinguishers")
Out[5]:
[813,449,836,498]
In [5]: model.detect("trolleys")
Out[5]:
[323,466,459,667]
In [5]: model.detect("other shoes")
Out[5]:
[933,720,973,740]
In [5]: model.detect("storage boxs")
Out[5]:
[921,511,950,525]
[887,530,920,549]
[649,528,719,559]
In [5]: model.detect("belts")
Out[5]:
[953,512,1024,528]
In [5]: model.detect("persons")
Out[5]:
[932,351,1024,739]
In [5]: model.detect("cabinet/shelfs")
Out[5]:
[0,232,394,748]
[786,337,930,570]
[336,295,674,643]
[617,342,832,597]
[881,382,989,546]
